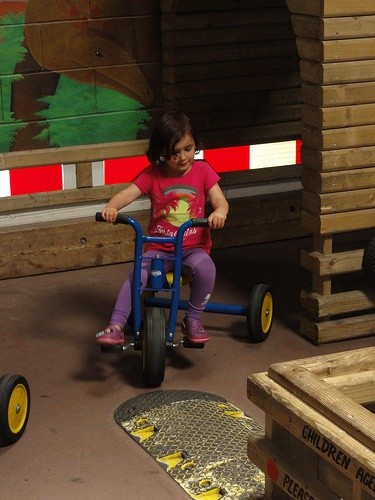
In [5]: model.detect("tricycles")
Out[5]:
[94,211,275,389]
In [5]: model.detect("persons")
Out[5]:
[95,110,229,347]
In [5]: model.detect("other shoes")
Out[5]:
[94,322,125,345]
[181,317,210,344]
